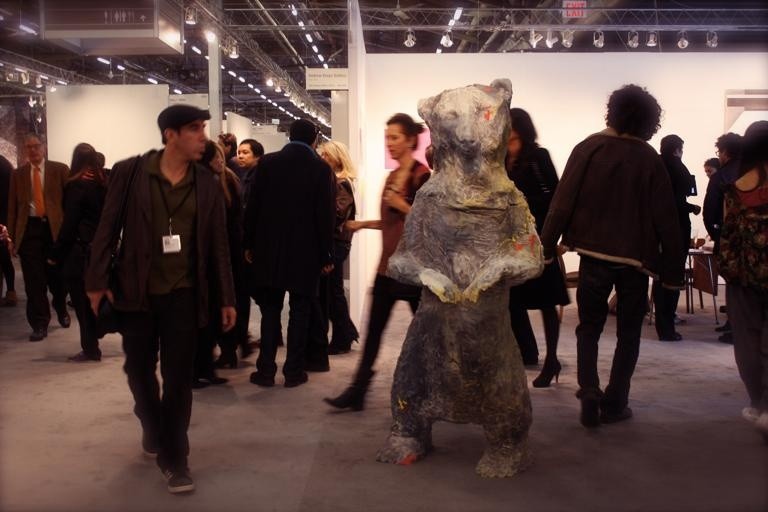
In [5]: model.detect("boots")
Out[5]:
[323,367,374,410]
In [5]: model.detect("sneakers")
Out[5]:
[157,460,195,493]
[601,407,632,422]
[284,372,307,387]
[580,392,600,427]
[6,291,17,305]
[742,407,768,430]
[141,429,160,457]
[250,372,274,387]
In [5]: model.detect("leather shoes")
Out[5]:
[30,327,47,340]
[68,350,100,362]
[659,330,682,341]
[192,344,253,389]
[52,300,70,327]
[716,322,734,344]
[674,316,688,325]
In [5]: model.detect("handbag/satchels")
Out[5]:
[84,241,132,327]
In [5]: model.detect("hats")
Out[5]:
[158,105,211,128]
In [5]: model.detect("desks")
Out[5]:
[649,247,719,326]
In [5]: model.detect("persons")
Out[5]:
[702,120,768,439]
[504,107,571,388]
[82,103,237,493]
[654,135,701,341]
[324,112,431,412]
[0,136,123,362]
[209,119,359,389]
[540,84,688,426]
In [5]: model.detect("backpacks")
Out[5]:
[717,204,768,288]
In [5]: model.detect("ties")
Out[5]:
[34,167,45,218]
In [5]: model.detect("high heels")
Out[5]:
[533,359,560,386]
[330,337,359,355]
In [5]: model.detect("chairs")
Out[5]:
[555,245,580,323]
[680,238,705,312]
